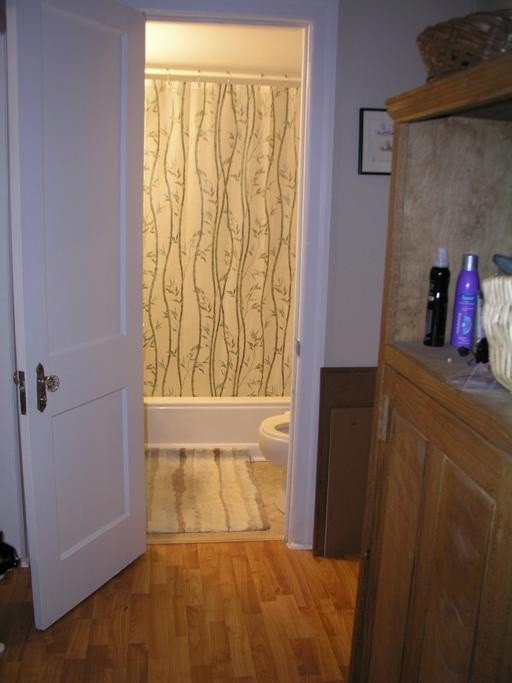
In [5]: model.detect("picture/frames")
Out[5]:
[358,106,395,176]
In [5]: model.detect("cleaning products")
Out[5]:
[452,253,480,348]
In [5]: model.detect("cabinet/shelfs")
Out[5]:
[338,48,511,682]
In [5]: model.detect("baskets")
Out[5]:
[416,8,512,82]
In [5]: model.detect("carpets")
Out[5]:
[144,444,271,534]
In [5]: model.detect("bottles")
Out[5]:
[450,253,477,347]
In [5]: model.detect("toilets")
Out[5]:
[257,410,291,470]
